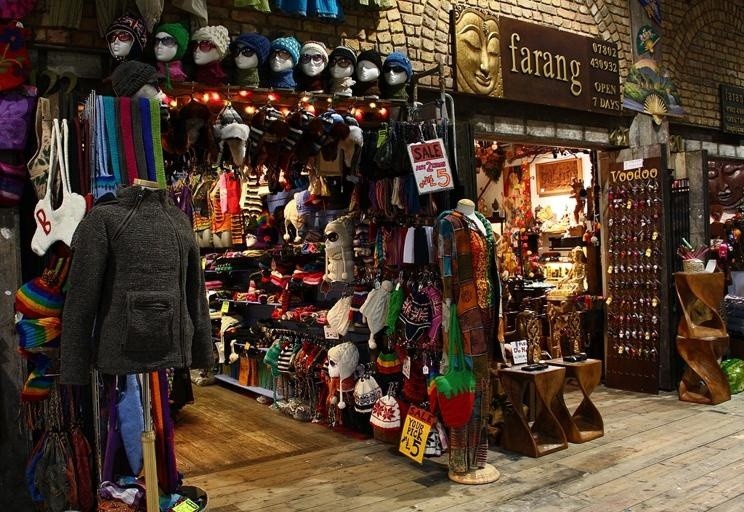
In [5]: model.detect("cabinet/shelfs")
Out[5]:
[216,296,281,403]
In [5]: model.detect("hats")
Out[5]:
[386,277,403,362]
[152,23,189,92]
[281,189,316,245]
[353,374,384,414]
[323,210,360,281]
[272,35,303,70]
[265,334,328,422]
[422,284,445,344]
[395,287,432,360]
[191,23,231,64]
[326,296,356,337]
[168,97,219,163]
[338,112,365,168]
[248,102,286,166]
[208,102,250,167]
[279,109,318,152]
[369,393,403,433]
[332,43,358,68]
[111,61,157,98]
[302,40,330,68]
[357,48,383,74]
[293,108,350,163]
[326,342,359,410]
[232,31,272,68]
[382,52,413,79]
[374,347,402,384]
[357,280,394,351]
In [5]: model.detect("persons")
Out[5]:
[456,7,500,96]
[708,156,744,210]
[230,33,269,90]
[324,215,353,249]
[357,49,383,100]
[382,51,412,103]
[269,36,299,92]
[328,45,357,99]
[151,23,188,80]
[328,342,359,378]
[192,25,230,87]
[300,42,328,94]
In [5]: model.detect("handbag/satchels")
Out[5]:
[432,301,475,429]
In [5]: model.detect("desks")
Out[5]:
[676,334,732,405]
[547,357,604,442]
[499,361,568,456]
[674,272,727,338]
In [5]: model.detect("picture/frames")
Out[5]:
[501,164,530,228]
[536,159,581,197]
